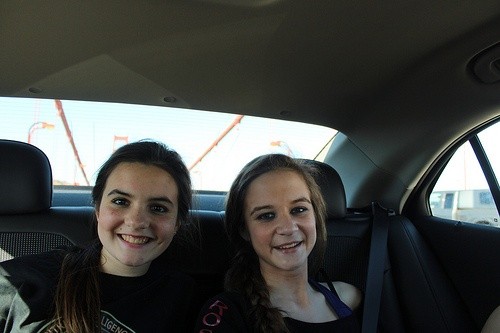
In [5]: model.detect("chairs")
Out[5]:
[1,138,89,263]
[174,156,457,333]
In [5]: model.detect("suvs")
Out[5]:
[430,188,499,227]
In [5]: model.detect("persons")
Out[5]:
[1,142,211,333]
[214,155,388,333]
